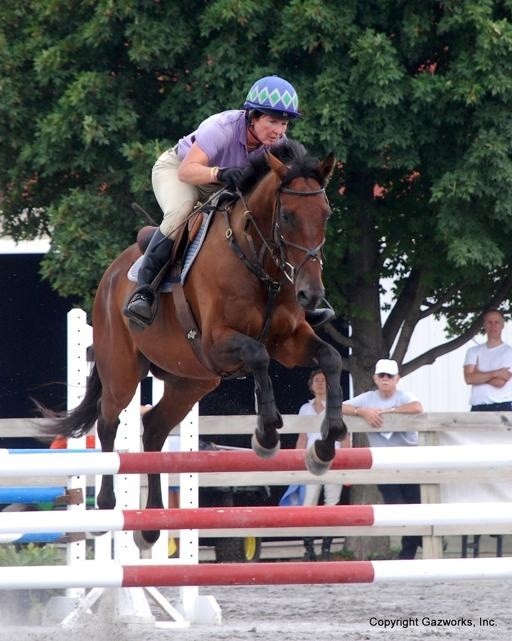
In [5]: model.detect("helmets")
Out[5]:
[244,74,302,122]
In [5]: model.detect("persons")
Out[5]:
[295,358,423,560]
[129,74,336,327]
[463,309,512,411]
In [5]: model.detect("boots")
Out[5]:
[304,536,333,561]
[127,225,177,333]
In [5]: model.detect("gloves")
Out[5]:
[216,165,249,191]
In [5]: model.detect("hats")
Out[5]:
[374,359,399,376]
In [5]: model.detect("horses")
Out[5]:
[23,142,340,555]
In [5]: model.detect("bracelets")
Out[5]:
[211,166,218,183]
